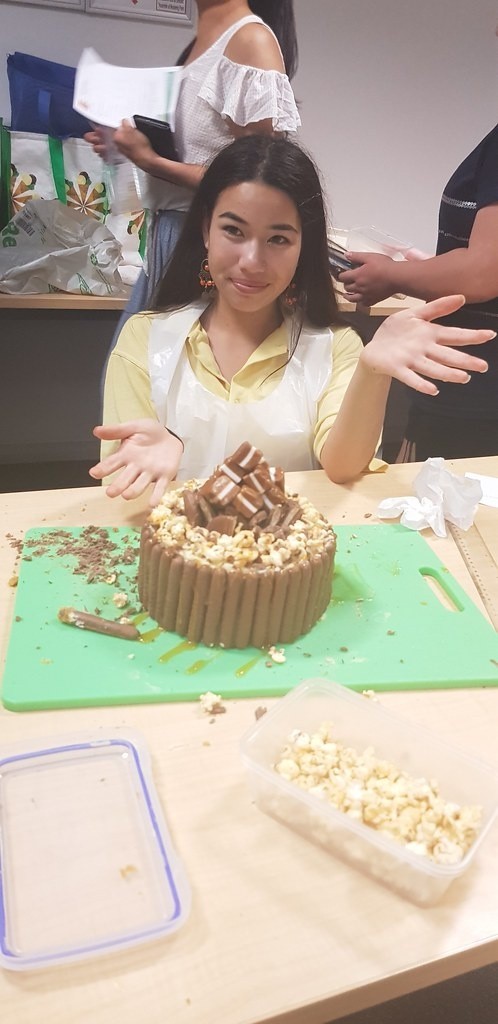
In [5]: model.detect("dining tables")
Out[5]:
[0,452,497,1022]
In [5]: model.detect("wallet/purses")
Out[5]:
[133,114,180,163]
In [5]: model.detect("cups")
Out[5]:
[100,120,154,216]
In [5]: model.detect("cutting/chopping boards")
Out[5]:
[1,520,494,714]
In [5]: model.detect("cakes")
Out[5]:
[134,441,339,650]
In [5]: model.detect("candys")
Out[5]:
[57,608,141,643]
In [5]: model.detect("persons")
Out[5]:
[85,0,302,402]
[88,131,496,510]
[338,119,496,466]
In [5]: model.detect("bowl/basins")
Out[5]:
[237,678,498,907]
[346,224,414,262]
